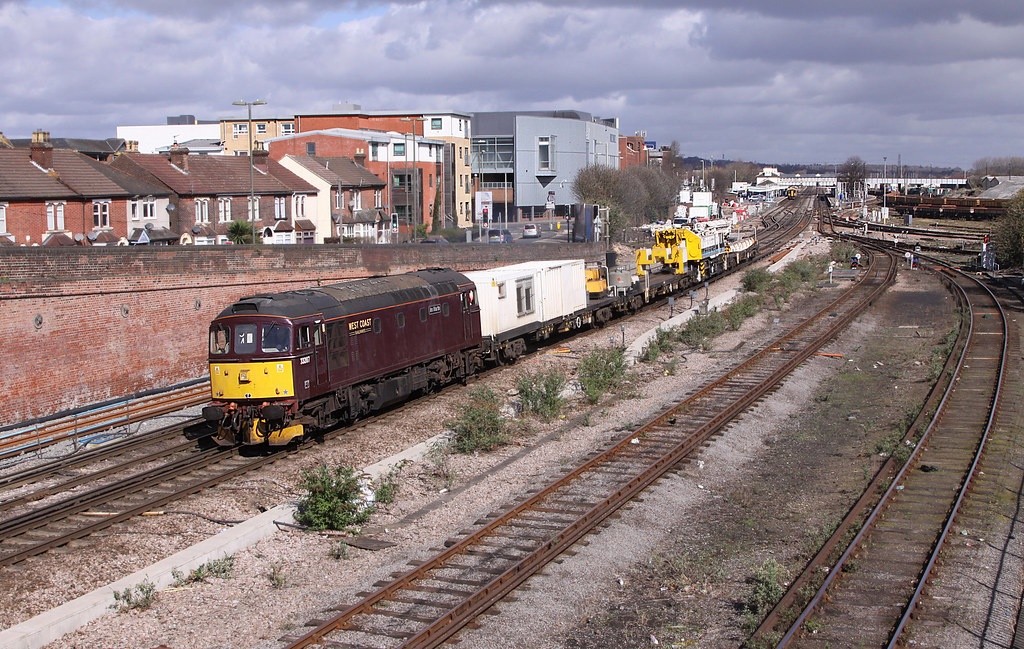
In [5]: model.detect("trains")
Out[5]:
[201,212,760,453]
[787,184,800,201]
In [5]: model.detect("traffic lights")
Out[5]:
[391,212,399,234]
[564,204,570,220]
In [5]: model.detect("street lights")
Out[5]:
[481,149,487,190]
[883,156,887,224]
[232,99,267,243]
[400,116,429,243]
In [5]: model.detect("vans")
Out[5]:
[523,223,541,238]
[487,230,514,244]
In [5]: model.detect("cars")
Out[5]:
[420,234,448,243]
[748,194,766,202]
